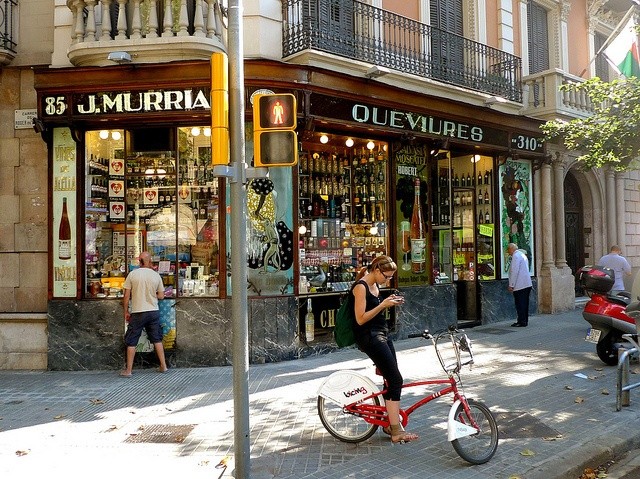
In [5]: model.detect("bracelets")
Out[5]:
[377,306,383,311]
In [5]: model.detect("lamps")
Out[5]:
[107,51,133,64]
[484,96,508,106]
[364,65,389,79]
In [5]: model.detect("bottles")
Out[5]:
[165,191,171,203]
[325,273,332,291]
[445,192,449,206]
[352,148,358,168]
[368,149,374,164]
[360,146,367,165]
[456,191,460,205]
[159,191,165,205]
[472,173,476,187]
[331,195,336,218]
[484,187,489,204]
[484,168,488,184]
[57,195,73,262]
[301,199,330,218]
[95,157,109,175]
[461,173,466,188]
[127,205,133,221]
[410,176,429,276]
[467,172,471,188]
[467,191,471,206]
[301,174,348,195]
[478,209,484,225]
[301,151,344,174]
[200,200,206,219]
[478,170,483,187]
[305,298,314,343]
[439,174,454,186]
[440,206,450,225]
[91,176,108,198]
[478,188,483,205]
[194,201,198,220]
[455,174,459,187]
[377,165,384,183]
[354,167,376,223]
[377,142,384,164]
[172,189,177,203]
[89,153,94,174]
[379,184,384,201]
[456,239,474,252]
[461,191,466,206]
[342,149,349,167]
[485,209,490,224]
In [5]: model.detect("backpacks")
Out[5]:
[333,280,369,347]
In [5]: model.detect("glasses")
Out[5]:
[507,248,511,250]
[378,267,393,280]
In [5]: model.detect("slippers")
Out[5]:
[119,371,132,376]
[160,368,169,372]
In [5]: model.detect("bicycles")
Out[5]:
[315,324,499,466]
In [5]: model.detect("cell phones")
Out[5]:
[392,295,404,303]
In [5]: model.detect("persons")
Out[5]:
[120,251,168,376]
[508,243,533,327]
[348,254,418,442]
[599,244,632,291]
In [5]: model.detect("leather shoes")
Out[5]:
[511,323,526,327]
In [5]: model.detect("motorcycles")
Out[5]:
[574,264,640,367]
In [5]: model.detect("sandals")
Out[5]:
[390,421,421,444]
[383,419,392,435]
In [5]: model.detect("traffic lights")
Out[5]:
[253,93,298,168]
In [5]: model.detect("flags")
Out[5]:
[579,3,640,81]
